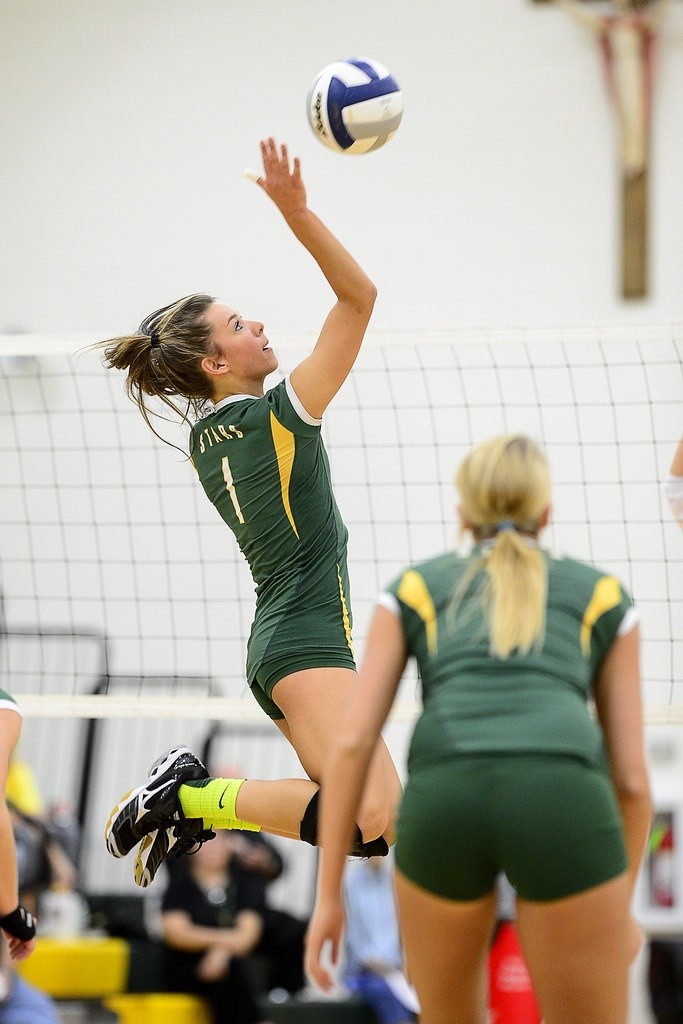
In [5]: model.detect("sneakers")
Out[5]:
[134,816,217,888]
[105,746,210,858]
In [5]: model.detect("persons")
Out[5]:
[301,432,653,1024]
[1,688,420,1024]
[103,134,402,895]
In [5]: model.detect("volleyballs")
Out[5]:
[305,55,406,156]
